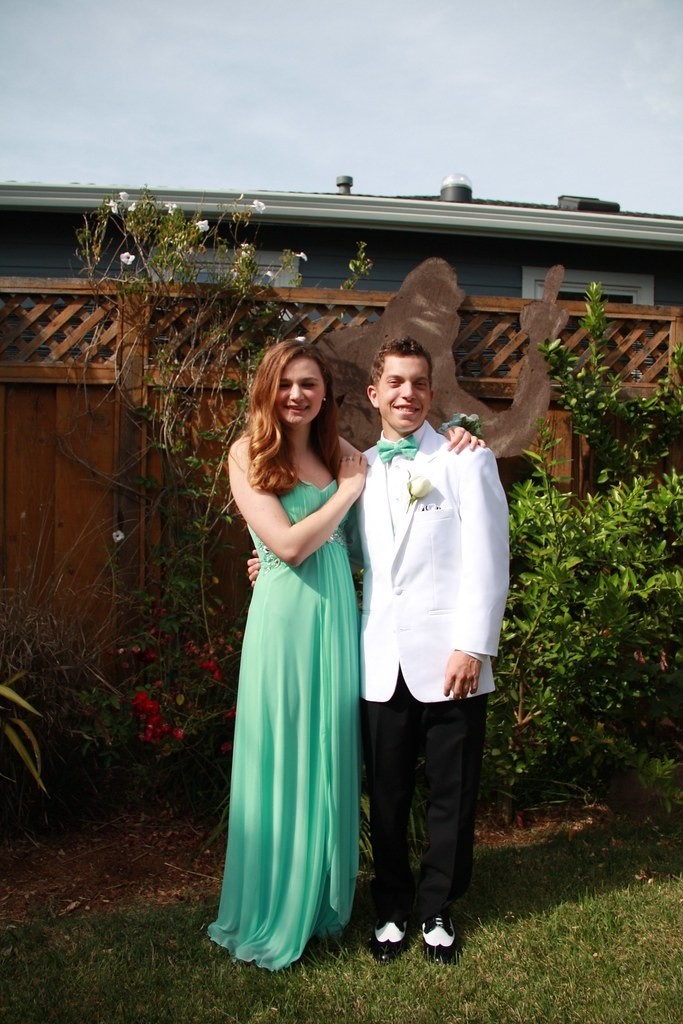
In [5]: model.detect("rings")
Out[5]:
[341,456,352,461]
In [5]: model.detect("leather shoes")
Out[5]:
[371,915,407,964]
[421,912,455,963]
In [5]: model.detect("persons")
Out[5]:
[204,340,486,970]
[248,336,509,965]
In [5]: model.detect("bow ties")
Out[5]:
[376,434,417,462]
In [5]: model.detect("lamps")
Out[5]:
[441,172,474,203]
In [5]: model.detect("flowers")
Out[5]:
[406,475,433,512]
